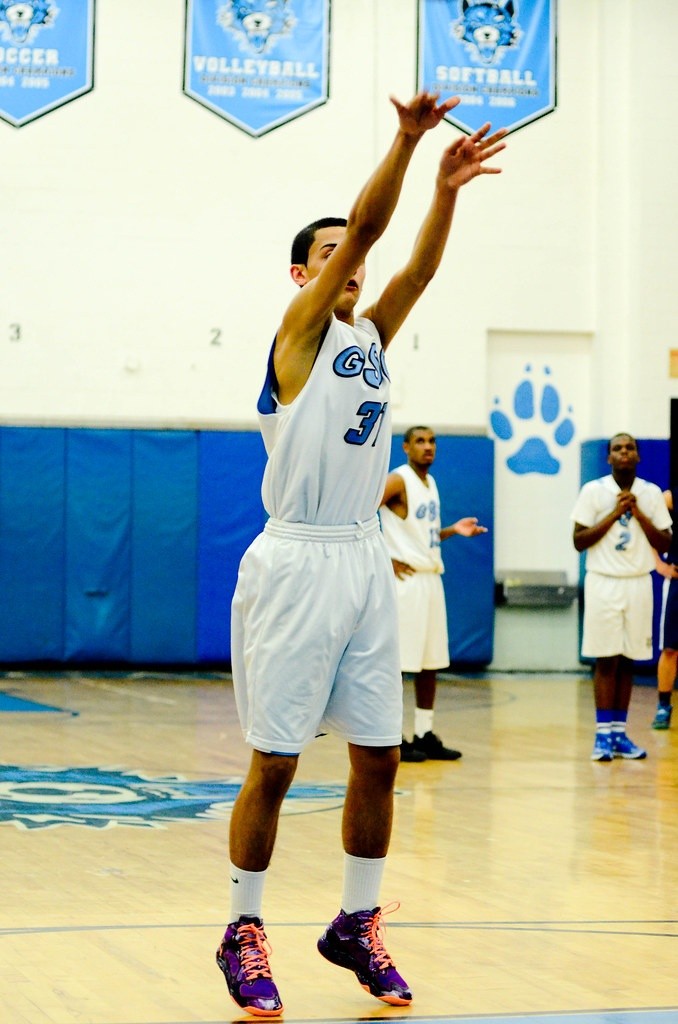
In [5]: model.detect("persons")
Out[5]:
[215,90,508,1016]
[574,434,678,760]
[377,426,489,762]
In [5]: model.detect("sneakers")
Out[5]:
[318,899,413,1006]
[399,738,424,762]
[652,703,673,729]
[609,730,647,760]
[215,918,284,1017]
[413,731,462,762]
[590,731,614,762]
